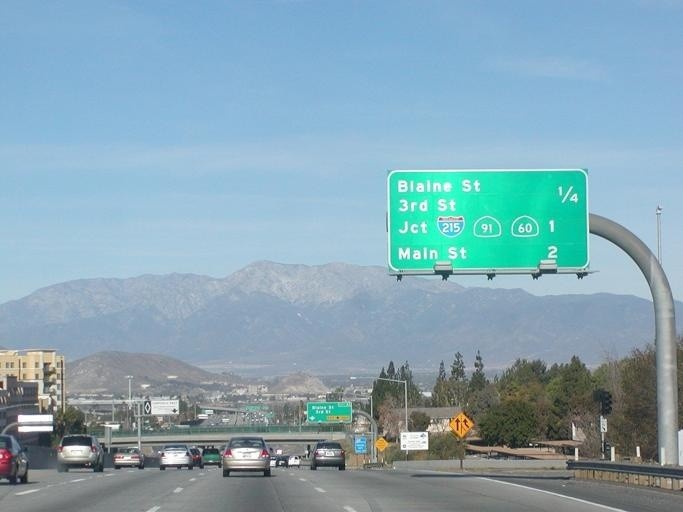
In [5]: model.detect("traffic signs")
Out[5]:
[354,435,367,454]
[143,400,179,415]
[385,169,589,276]
[307,402,353,424]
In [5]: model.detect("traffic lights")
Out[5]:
[602,392,612,416]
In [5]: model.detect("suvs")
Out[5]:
[56,433,105,472]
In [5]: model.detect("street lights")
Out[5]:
[350,376,408,460]
[125,375,134,409]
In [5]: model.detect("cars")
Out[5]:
[0,434,30,483]
[201,448,222,468]
[223,436,271,476]
[113,447,145,469]
[276,456,300,468]
[309,441,345,470]
[158,444,201,470]
